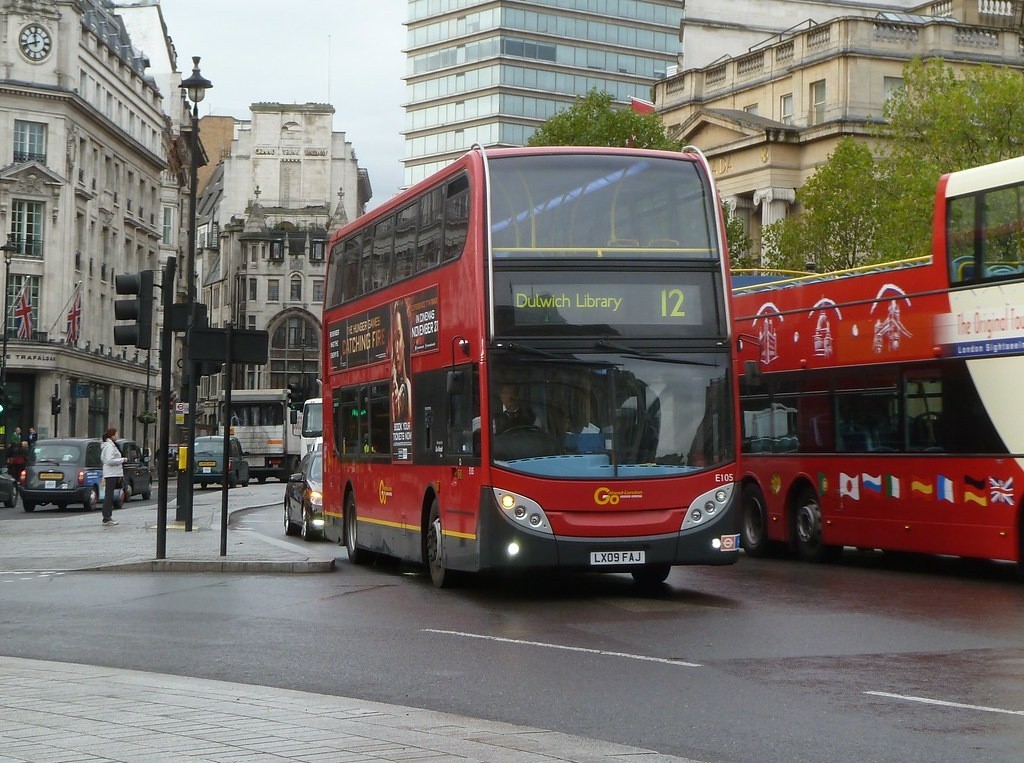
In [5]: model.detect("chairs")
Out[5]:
[650,238,679,247]
[738,254,1016,296]
[751,411,941,455]
[608,239,638,248]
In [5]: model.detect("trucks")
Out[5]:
[216,388,324,485]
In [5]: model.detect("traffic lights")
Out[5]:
[0,386,6,416]
[113,270,152,349]
[286,382,305,413]
[51,397,61,416]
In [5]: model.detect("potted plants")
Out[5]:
[138,410,157,424]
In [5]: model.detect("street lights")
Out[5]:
[1,234,16,467]
[179,54,213,532]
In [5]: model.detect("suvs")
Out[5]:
[113,439,153,502]
[20,436,124,515]
[192,435,251,488]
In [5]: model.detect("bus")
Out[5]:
[726,152,1024,583]
[290,397,323,462]
[321,141,765,590]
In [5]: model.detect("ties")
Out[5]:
[505,409,518,419]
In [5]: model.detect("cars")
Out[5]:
[283,450,325,541]
[153,444,182,483]
[0,469,19,508]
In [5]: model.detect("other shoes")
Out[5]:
[103,518,118,525]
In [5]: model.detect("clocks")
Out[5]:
[20,26,49,59]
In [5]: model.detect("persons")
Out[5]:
[493,380,542,432]
[7,427,38,482]
[155,448,179,475]
[100,428,128,525]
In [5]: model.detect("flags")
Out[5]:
[15,281,34,340]
[66,290,83,342]
